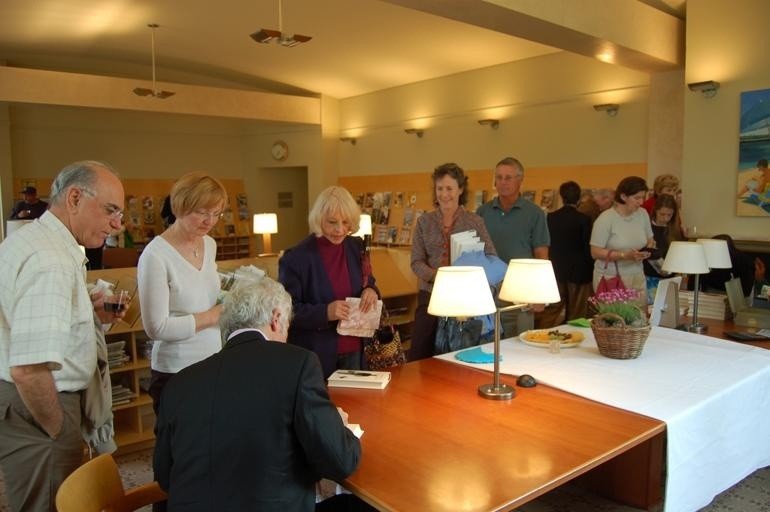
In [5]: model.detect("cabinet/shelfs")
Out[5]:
[212,235,252,261]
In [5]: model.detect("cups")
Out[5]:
[103,288,129,313]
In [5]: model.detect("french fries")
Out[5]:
[525,328,549,341]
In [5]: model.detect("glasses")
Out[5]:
[81,189,124,218]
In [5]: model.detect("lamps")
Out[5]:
[340,133,360,148]
[403,124,428,140]
[131,23,176,101]
[248,2,313,50]
[686,78,722,100]
[592,101,623,118]
[252,211,282,257]
[659,238,733,334]
[475,115,503,133]
[425,256,561,401]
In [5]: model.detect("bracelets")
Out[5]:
[621,252,624,260]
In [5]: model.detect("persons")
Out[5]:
[537,181,592,329]
[640,173,685,305]
[9,184,49,220]
[0,161,126,511]
[136,170,227,512]
[411,163,509,360]
[589,176,649,302]
[278,184,380,384]
[152,277,373,511]
[475,157,551,342]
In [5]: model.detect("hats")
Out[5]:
[22,186,36,194]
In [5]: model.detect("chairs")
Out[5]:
[54,454,169,510]
[99,246,140,269]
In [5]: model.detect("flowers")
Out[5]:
[586,288,648,323]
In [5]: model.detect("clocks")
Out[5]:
[268,137,291,162]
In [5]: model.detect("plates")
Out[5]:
[520,329,583,347]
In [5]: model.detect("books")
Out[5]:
[105,337,155,408]
[643,287,732,321]
[327,370,391,389]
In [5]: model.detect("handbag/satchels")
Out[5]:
[597,274,626,295]
[363,332,405,370]
[434,319,482,355]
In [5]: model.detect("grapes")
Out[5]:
[548,328,571,341]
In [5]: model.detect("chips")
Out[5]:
[565,331,586,344]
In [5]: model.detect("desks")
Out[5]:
[323,304,769,511]
[85,243,423,456]
